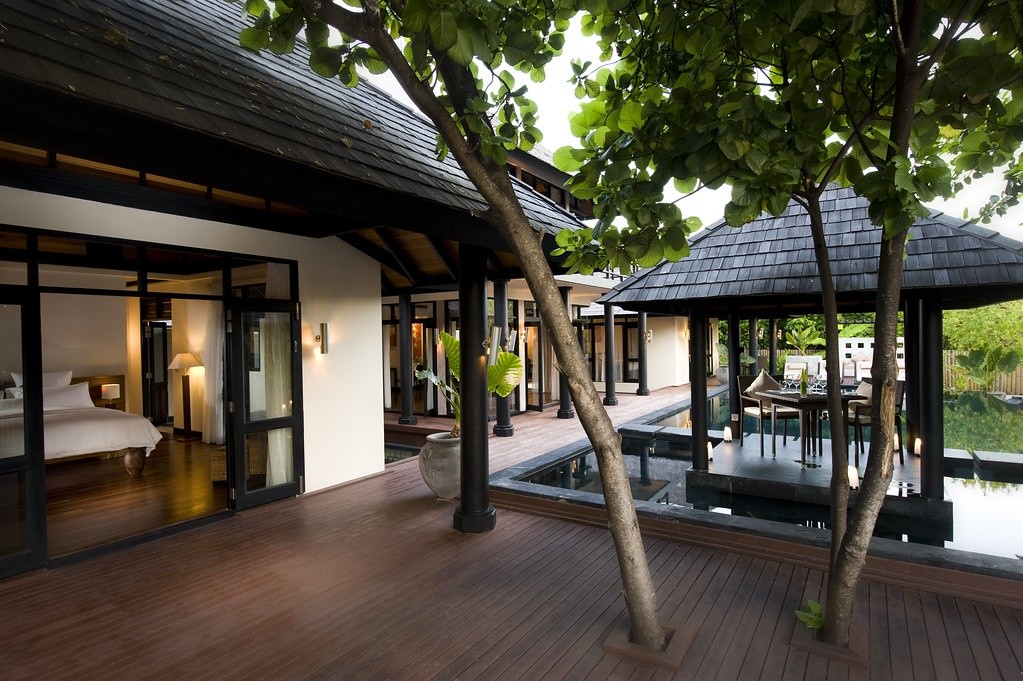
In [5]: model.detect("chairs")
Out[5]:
[818,377,905,468]
[737,375,810,457]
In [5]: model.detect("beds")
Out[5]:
[0,407,163,475]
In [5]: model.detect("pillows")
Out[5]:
[848,381,872,415]
[5,381,95,411]
[743,369,783,408]
[10,371,72,388]
[0,397,23,417]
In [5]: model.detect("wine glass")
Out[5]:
[779,379,787,395]
[806,375,827,395]
[786,373,801,392]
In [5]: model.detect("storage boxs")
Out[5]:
[211,451,227,481]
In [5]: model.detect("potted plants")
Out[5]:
[716,343,756,384]
[415,331,522,499]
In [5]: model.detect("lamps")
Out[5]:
[914,438,921,456]
[316,322,328,354]
[894,434,899,452]
[848,465,859,490]
[724,426,732,443]
[101,383,120,409]
[168,353,203,442]
[708,442,713,461]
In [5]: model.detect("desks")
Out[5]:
[755,392,868,469]
